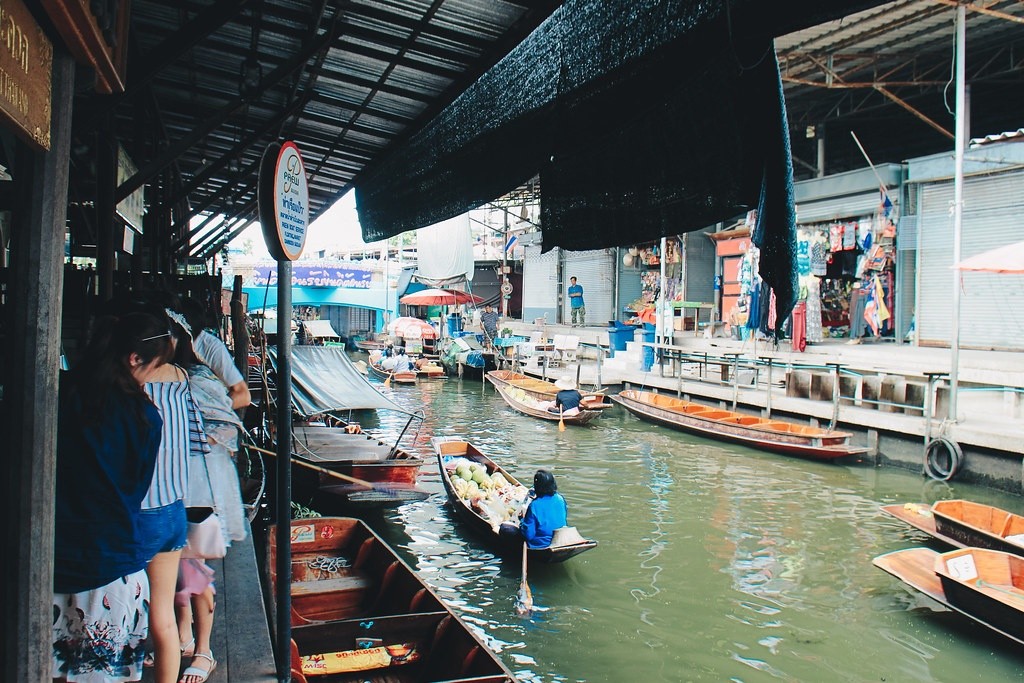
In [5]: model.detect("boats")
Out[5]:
[871,546,1024,645]
[298,319,341,345]
[430,435,600,563]
[392,347,445,376]
[487,370,614,411]
[608,390,874,458]
[495,385,604,425]
[265,345,425,508]
[369,356,417,383]
[880,500,1024,558]
[266,516,521,683]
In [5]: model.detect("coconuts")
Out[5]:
[450,465,506,488]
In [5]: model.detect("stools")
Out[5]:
[698,321,728,339]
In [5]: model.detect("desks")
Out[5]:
[655,302,716,339]
[494,337,521,355]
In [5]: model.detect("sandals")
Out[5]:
[142,638,195,668]
[177,648,218,683]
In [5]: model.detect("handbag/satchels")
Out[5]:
[177,509,228,560]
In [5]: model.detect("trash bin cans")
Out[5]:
[642,322,660,371]
[606,319,636,358]
[447,313,463,336]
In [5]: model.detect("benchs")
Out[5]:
[300,640,430,676]
[276,575,385,622]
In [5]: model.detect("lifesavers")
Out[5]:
[921,434,964,479]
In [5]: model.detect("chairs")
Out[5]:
[515,332,542,355]
[552,335,566,360]
[556,336,581,360]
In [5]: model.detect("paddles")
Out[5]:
[384,372,393,384]
[559,404,564,431]
[520,541,534,609]
[241,440,396,494]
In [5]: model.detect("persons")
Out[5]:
[0,282,251,683]
[548,377,589,415]
[480,303,501,345]
[567,277,586,328]
[500,470,568,548]
[376,344,429,372]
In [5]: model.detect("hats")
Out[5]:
[417,353,425,358]
[622,253,634,267]
[554,375,578,391]
[629,246,641,256]
[545,525,590,550]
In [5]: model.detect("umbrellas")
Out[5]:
[745,276,783,343]
[384,316,438,340]
[400,284,487,338]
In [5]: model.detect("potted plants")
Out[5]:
[502,327,513,338]
